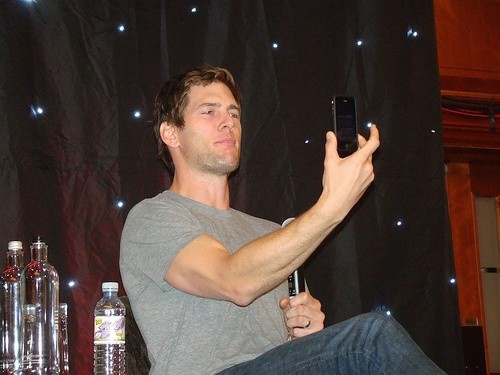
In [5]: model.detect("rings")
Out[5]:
[304,319,311,329]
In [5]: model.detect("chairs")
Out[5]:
[117,296,152,375]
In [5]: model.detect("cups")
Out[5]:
[20,303,69,375]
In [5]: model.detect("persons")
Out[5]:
[118,66,448,375]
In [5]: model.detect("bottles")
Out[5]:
[93,282,127,375]
[0,240,35,375]
[20,235,60,375]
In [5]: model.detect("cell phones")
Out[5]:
[332,95,359,159]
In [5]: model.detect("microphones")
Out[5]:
[282,218,305,299]
